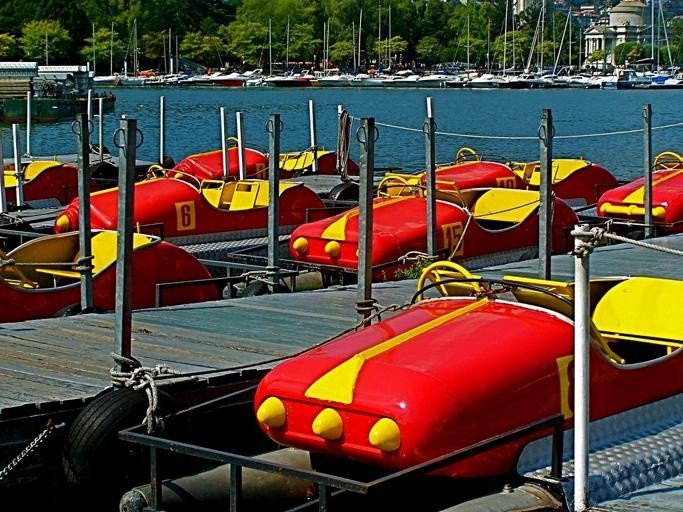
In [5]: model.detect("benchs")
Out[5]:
[1,144,592,292]
[589,276,683,356]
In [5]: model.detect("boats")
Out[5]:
[0,59,117,124]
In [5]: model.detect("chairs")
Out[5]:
[500,274,626,366]
[421,265,498,299]
